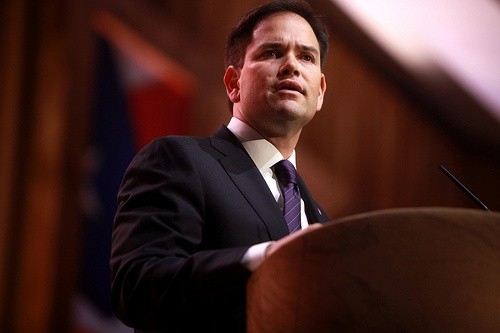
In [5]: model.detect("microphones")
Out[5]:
[440,164,490,212]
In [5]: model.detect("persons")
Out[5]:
[109,0,330,333]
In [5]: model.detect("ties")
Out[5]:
[274,159,302,234]
